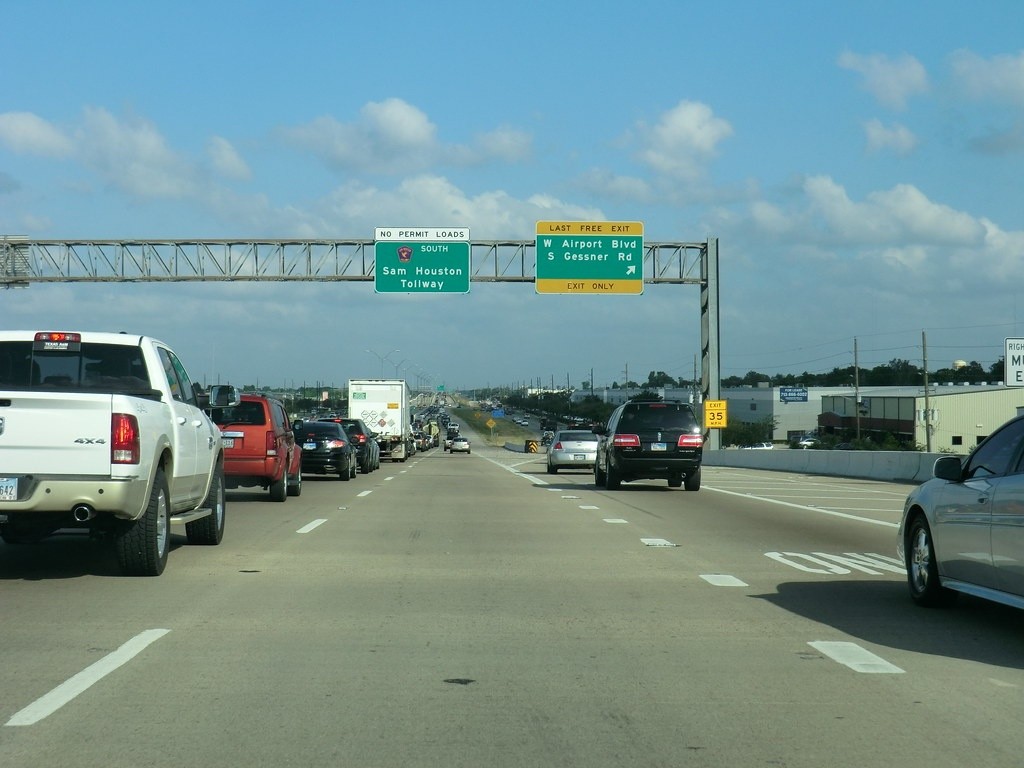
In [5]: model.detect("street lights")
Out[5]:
[386,357,433,392]
[364,348,400,377]
[827,393,860,439]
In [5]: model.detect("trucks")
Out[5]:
[347,378,415,463]
[539,416,558,432]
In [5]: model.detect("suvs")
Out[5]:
[317,418,380,474]
[790,433,811,444]
[743,441,774,449]
[591,397,704,491]
[0,329,241,575]
[195,393,305,502]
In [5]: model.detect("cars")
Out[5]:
[479,399,531,427]
[544,430,603,474]
[540,430,555,447]
[293,420,359,482]
[310,407,346,419]
[898,414,1023,612]
[409,399,472,454]
[798,438,826,448]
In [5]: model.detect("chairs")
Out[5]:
[14,357,42,387]
[100,354,133,378]
